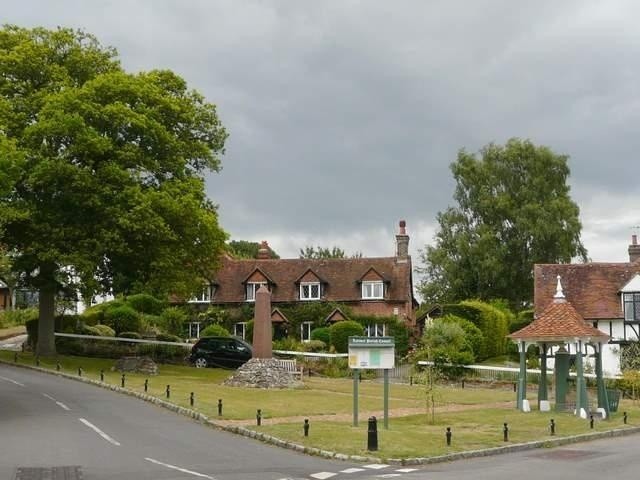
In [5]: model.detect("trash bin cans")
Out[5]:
[607,390,620,412]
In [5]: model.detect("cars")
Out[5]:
[408,345,417,354]
[190,336,253,373]
